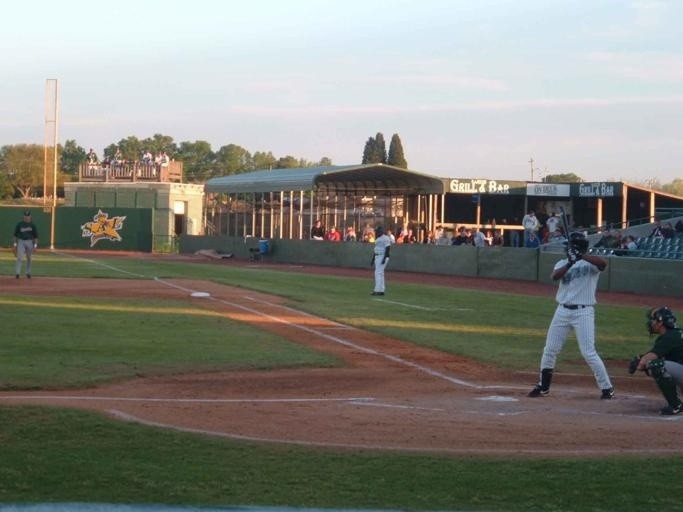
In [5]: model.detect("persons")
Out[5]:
[86,148,170,166]
[528,233,615,399]
[14,210,39,278]
[369,226,391,296]
[628,306,683,415]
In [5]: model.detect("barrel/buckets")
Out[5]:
[257,240,269,255]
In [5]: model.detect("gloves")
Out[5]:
[567,247,581,264]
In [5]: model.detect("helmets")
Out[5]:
[646,306,676,329]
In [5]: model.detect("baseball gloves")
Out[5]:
[628,354,642,375]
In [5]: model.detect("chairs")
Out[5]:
[583,221,683,261]
[247,238,269,264]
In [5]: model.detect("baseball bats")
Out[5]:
[560,211,571,245]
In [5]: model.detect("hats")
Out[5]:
[562,232,589,246]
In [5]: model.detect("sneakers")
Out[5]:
[600,388,615,399]
[527,385,551,397]
[371,291,384,296]
[16,275,31,279]
[659,399,683,415]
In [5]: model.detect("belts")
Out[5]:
[561,302,586,310]
